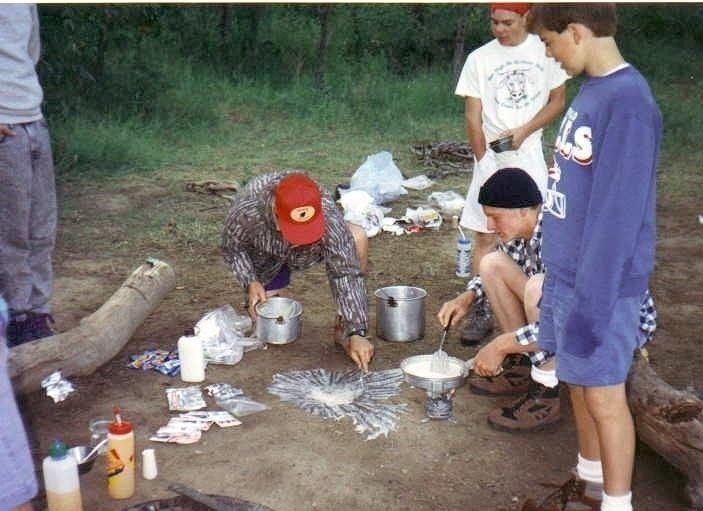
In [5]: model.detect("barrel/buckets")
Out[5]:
[374,270,427,343]
[255,297,303,345]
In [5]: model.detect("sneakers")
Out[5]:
[458,297,496,346]
[516,473,605,511]
[485,385,564,433]
[4,306,61,350]
[467,353,533,397]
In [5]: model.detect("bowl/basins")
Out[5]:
[253,298,303,344]
[64,446,97,475]
[488,135,514,153]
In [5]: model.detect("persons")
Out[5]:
[0,296,44,511]
[222,168,375,374]
[436,167,658,435]
[454,4,574,345]
[0,3,59,348]
[533,3,663,511]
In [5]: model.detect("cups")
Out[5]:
[88,415,117,457]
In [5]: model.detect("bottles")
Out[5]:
[178,326,204,382]
[107,401,136,502]
[41,442,82,511]
[456,227,473,277]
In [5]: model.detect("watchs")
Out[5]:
[347,329,368,337]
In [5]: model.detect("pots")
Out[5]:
[399,353,477,394]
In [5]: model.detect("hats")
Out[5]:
[275,172,326,247]
[477,166,543,208]
[489,3,530,15]
